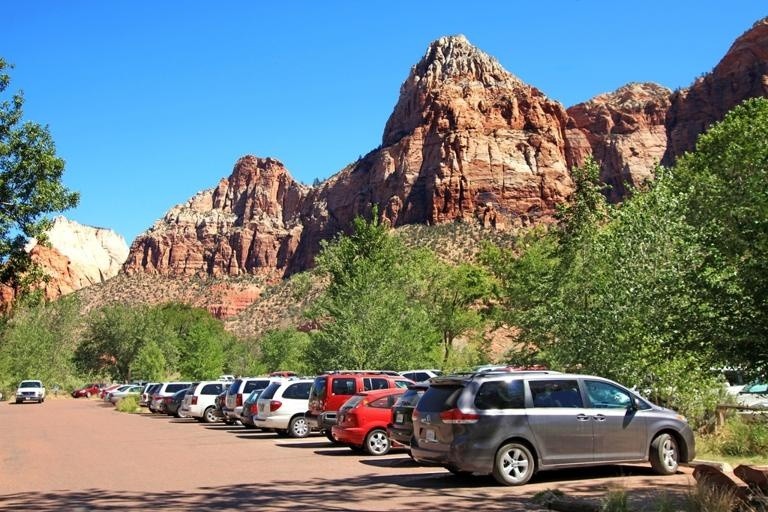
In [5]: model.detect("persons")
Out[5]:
[53,384,60,400]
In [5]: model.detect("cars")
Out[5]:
[71,379,142,405]
[331,389,406,455]
[16,378,45,403]
[399,363,551,391]
[628,365,755,402]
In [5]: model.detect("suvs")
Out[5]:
[410,370,696,483]
[302,369,418,442]
[139,371,316,437]
[387,376,429,453]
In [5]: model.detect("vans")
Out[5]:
[735,372,767,413]
